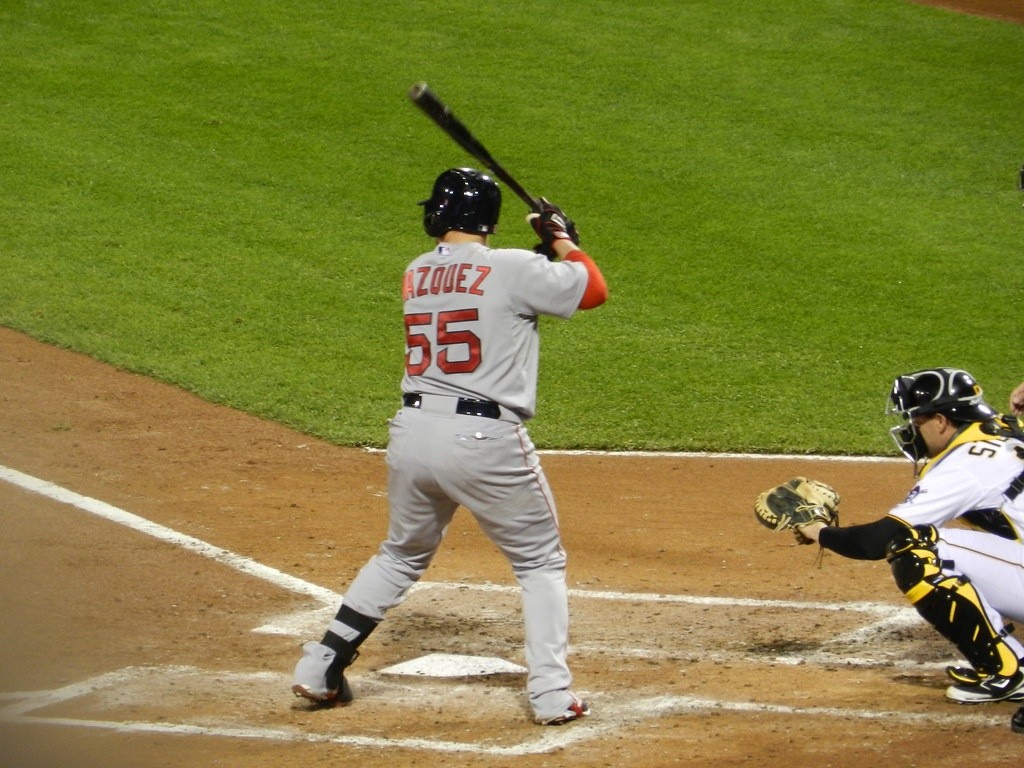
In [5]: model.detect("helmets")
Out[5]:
[416,167,502,237]
[892,366,998,423]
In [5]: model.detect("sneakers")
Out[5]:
[535,694,590,728]
[946,661,1024,705]
[291,641,360,708]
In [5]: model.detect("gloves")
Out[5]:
[534,220,578,261]
[524,196,571,246]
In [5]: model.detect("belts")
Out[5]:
[402,391,500,420]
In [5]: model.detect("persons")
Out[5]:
[753,368,1024,732]
[291,167,608,726]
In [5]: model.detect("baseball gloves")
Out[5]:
[754,475,842,547]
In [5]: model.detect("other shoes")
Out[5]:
[1011,705,1024,732]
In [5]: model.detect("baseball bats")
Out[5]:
[408,81,545,214]
